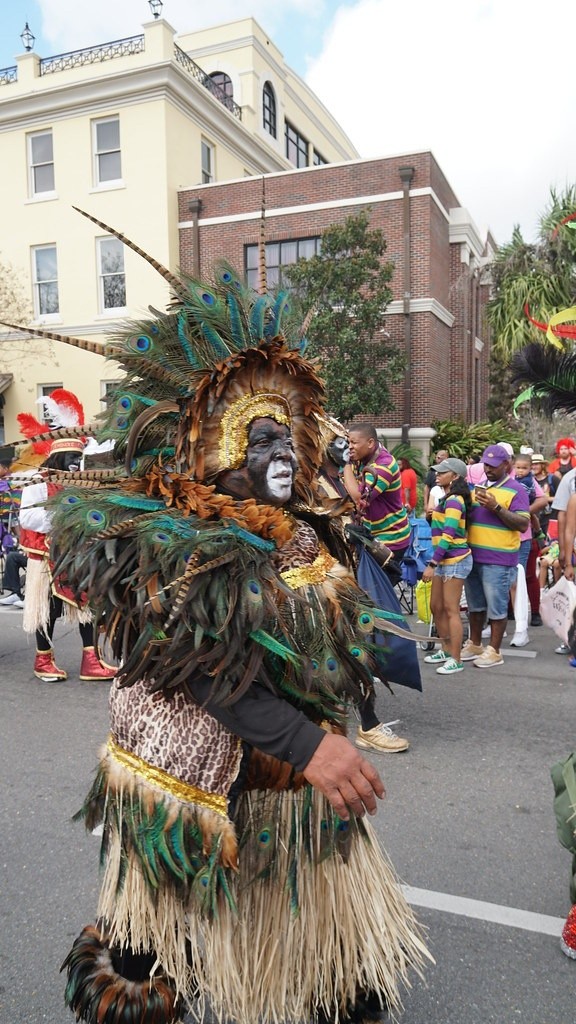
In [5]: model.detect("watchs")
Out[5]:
[492,504,502,514]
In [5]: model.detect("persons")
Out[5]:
[310,421,575,960]
[0,389,121,686]
[0,172,440,1024]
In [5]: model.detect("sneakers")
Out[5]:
[356,719,410,753]
[14,601,24,609]
[0,593,23,604]
[473,645,504,668]
[481,624,507,638]
[509,630,529,646]
[460,639,483,660]
[424,649,451,663]
[437,657,464,674]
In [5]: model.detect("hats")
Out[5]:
[48,438,85,457]
[479,445,510,467]
[497,442,513,458]
[430,458,468,478]
[532,454,549,465]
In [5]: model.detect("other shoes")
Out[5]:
[560,904,576,959]
[530,612,542,626]
[554,643,573,654]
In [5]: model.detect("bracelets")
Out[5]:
[429,563,437,568]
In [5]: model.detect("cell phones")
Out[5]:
[475,485,486,501]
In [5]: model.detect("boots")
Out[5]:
[34,648,67,682]
[80,646,119,680]
